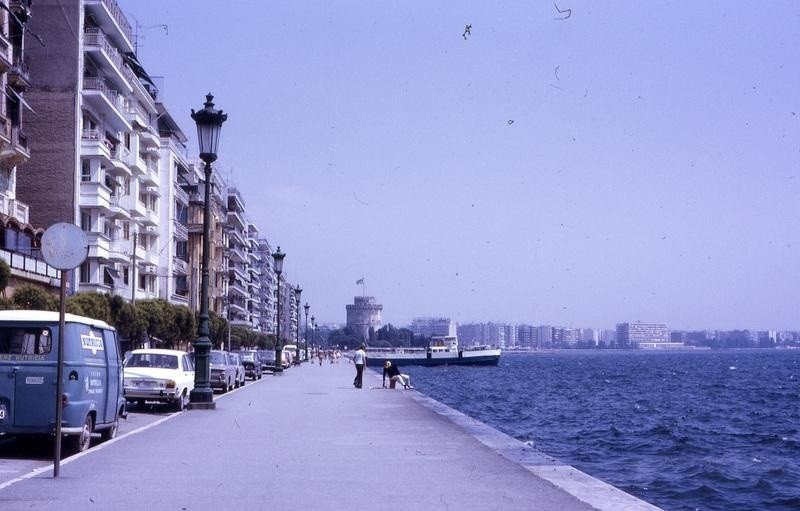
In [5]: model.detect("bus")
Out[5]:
[0,310,126,452]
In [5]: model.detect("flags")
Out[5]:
[356,278,364,284]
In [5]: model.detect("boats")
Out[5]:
[364,336,502,365]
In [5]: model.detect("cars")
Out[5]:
[238,350,264,382]
[209,350,236,392]
[228,352,247,388]
[123,349,195,412]
[260,350,277,371]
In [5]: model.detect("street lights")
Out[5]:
[293,284,303,364]
[191,92,228,409]
[303,301,310,361]
[271,246,286,372]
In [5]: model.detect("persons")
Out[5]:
[310,348,340,366]
[382,360,415,390]
[352,346,367,388]
